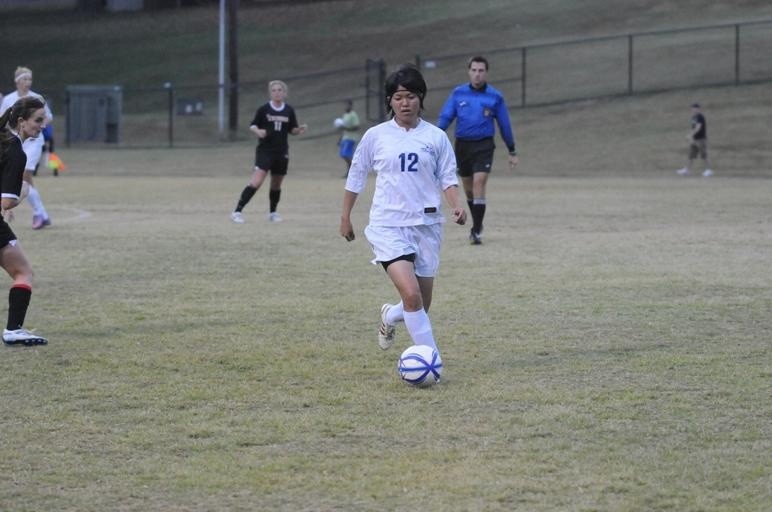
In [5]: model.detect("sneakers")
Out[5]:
[229,209,244,223]
[269,211,284,223]
[3,325,48,346]
[378,303,396,350]
[469,227,480,244]
[32,213,51,230]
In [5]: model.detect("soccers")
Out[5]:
[334,118,344,129]
[398,344,443,387]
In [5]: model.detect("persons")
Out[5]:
[0,66,53,230]
[677,103,714,177]
[231,81,308,223]
[437,55,519,244]
[339,67,468,357]
[32,123,59,176]
[340,98,360,178]
[0,97,50,345]
